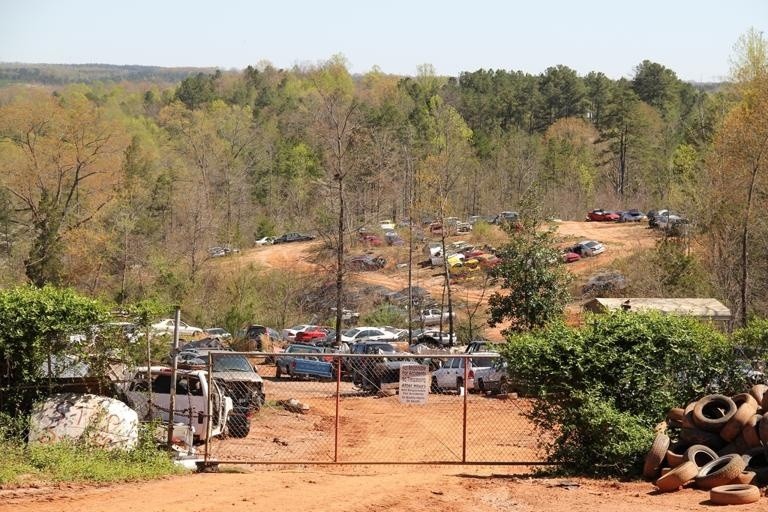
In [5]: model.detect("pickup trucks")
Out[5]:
[473,366,513,396]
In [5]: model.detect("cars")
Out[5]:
[243,323,491,384]
[352,211,519,285]
[586,208,681,226]
[254,232,316,246]
[563,240,605,263]
[92,318,234,344]
[208,246,239,258]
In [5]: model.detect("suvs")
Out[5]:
[124,352,234,446]
[177,347,266,412]
[430,352,508,395]
